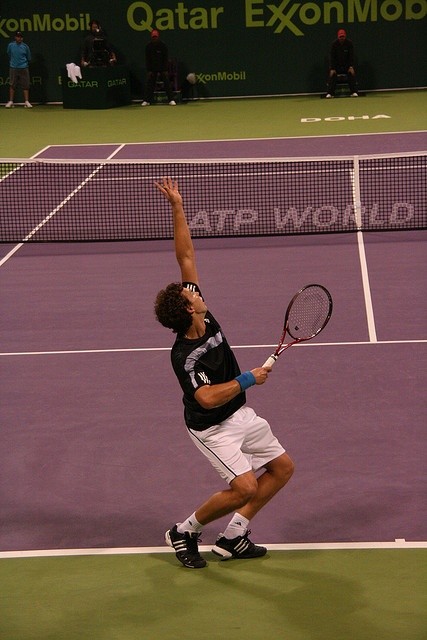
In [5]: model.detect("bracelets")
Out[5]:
[237,369,258,395]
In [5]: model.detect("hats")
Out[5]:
[338,29,346,37]
[15,32,24,37]
[151,29,159,38]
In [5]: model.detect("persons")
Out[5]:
[80,18,117,66]
[152,173,296,575]
[322,27,362,102]
[142,29,177,108]
[4,32,32,109]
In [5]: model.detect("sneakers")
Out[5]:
[5,101,15,108]
[326,94,331,98]
[210,528,267,559]
[352,93,358,97]
[164,522,207,568]
[24,101,33,108]
[141,101,150,106]
[169,101,176,106]
